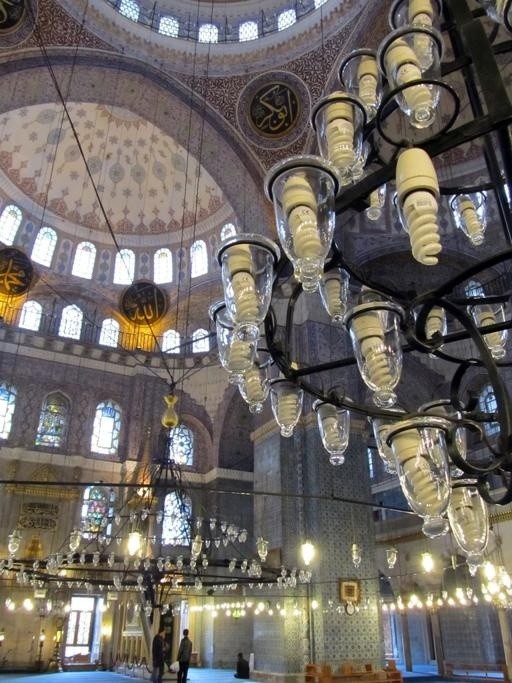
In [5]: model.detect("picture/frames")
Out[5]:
[339,579,361,603]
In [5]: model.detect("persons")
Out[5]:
[150,626,168,683]
[234,652,249,679]
[174,629,193,683]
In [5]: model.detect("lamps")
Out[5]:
[0,383,313,617]
[207,0,511,575]
